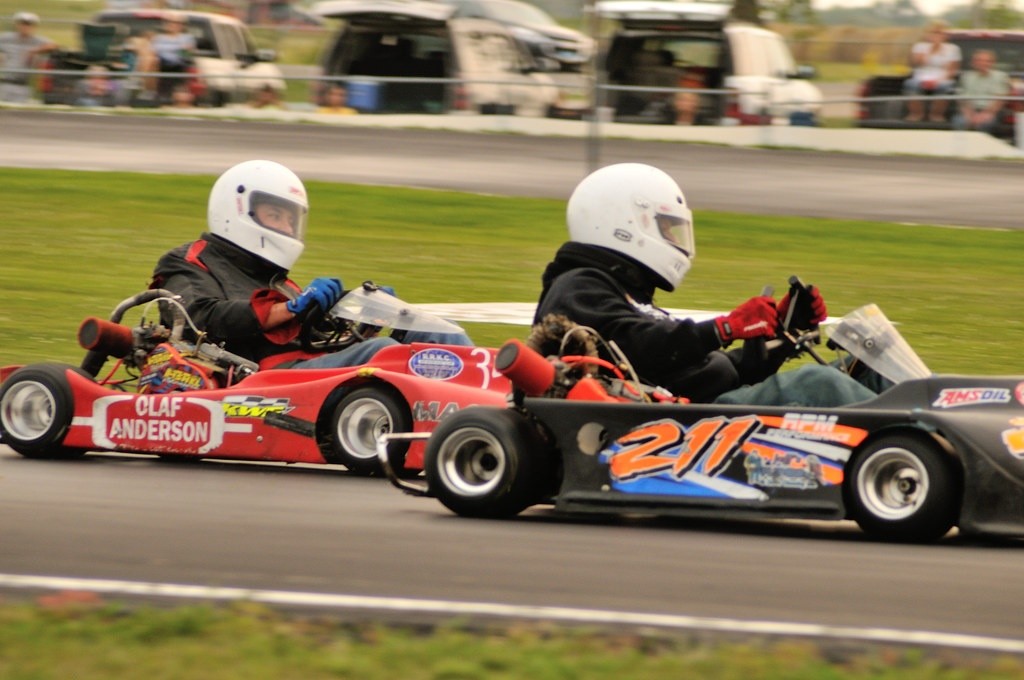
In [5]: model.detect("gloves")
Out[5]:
[715,296,778,348]
[364,287,396,330]
[287,277,343,313]
[776,284,827,330]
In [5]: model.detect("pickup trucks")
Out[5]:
[856,29,1024,143]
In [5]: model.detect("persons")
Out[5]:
[904,18,1010,134]
[0,10,357,115]
[534,163,894,407]
[660,72,716,124]
[149,160,474,387]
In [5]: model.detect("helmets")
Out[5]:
[565,162,696,292]
[206,160,309,272]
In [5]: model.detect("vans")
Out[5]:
[297,0,553,119]
[583,2,824,126]
[78,10,287,117]
